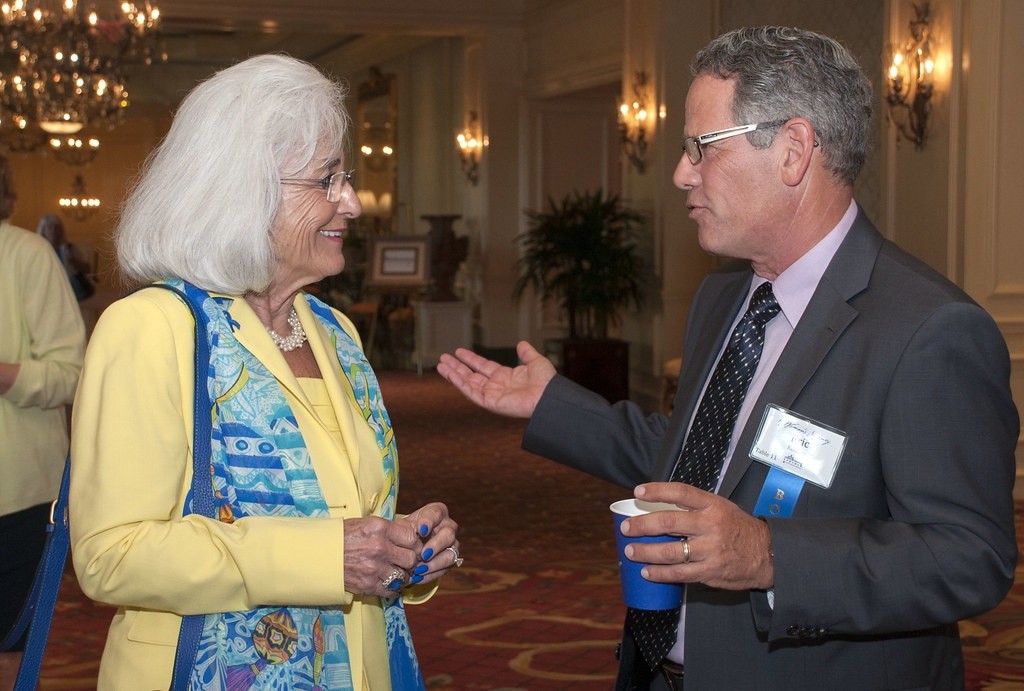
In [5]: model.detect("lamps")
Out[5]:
[884,0,940,155]
[454,110,483,186]
[0,0,171,224]
[612,70,652,176]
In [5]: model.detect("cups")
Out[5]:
[609,498,689,611]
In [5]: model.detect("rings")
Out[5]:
[446,545,464,567]
[381,565,406,592]
[680,538,690,563]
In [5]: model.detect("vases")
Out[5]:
[421,213,462,301]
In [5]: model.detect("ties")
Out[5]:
[628,282,782,672]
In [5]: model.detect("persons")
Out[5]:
[0,153,86,691]
[68,55,460,691]
[436,24,1020,691]
[38,215,84,303]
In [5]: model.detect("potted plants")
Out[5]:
[504,185,662,409]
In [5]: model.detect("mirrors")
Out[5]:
[354,72,398,218]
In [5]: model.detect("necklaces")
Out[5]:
[263,305,307,353]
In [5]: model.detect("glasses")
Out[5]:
[682,120,819,165]
[279,169,357,203]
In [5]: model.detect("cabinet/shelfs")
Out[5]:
[409,297,479,378]
